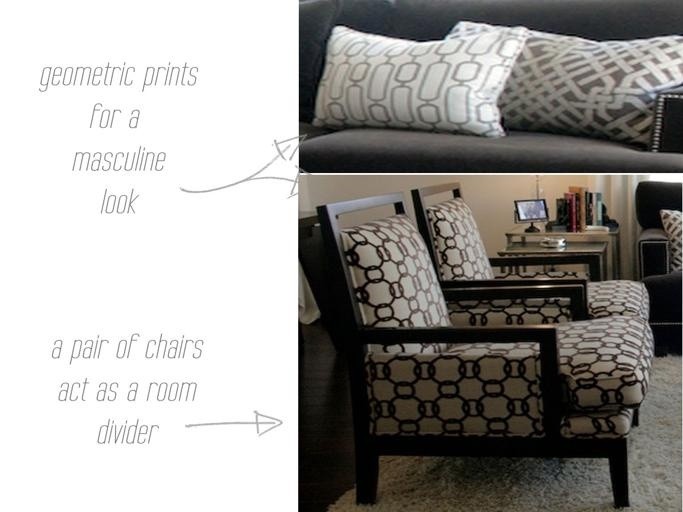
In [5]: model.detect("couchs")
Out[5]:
[299,1,683,171]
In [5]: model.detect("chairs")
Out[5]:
[316,180,656,510]
[634,180,682,358]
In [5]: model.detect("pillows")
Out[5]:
[445,17,683,149]
[310,23,532,138]
[660,206,682,273]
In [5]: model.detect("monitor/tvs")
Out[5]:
[513,198,548,221]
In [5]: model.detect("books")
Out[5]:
[556,186,612,232]
